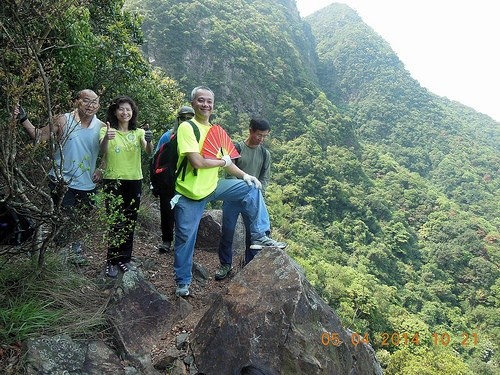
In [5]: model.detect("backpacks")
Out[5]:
[150,121,222,198]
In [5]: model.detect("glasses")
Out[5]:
[251,131,268,139]
[177,116,192,121]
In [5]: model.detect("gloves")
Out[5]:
[242,173,262,190]
[223,155,232,168]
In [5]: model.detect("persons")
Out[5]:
[98,96,153,277]
[156,106,195,252]
[14,89,107,249]
[173,86,287,297]
[215,118,272,279]
[144,123,153,142]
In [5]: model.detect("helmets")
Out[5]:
[178,106,195,117]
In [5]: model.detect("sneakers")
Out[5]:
[214,263,231,278]
[106,263,118,278]
[176,283,190,296]
[118,261,136,273]
[250,234,286,250]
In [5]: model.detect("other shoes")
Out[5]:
[72,243,81,253]
[159,242,171,252]
